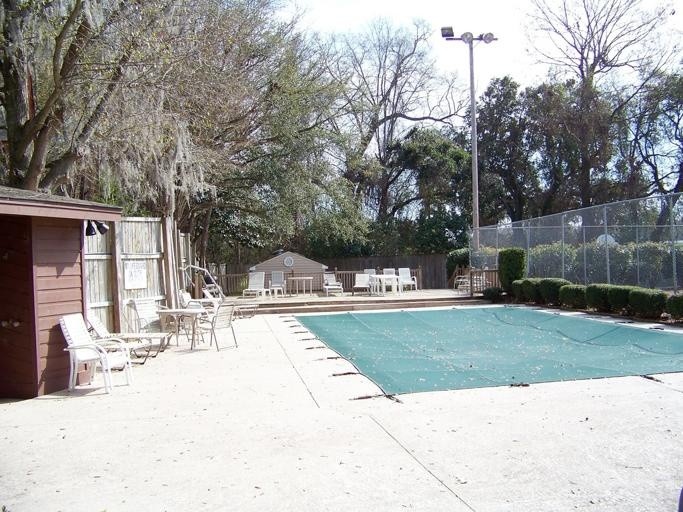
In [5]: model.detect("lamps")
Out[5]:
[85,219,110,236]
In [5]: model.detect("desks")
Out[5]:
[287,276,313,297]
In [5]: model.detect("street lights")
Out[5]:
[441,25,499,251]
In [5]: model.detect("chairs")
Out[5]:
[92,308,169,356]
[449,270,492,296]
[58,311,129,395]
[199,284,258,319]
[242,271,265,298]
[144,299,245,362]
[86,310,170,365]
[320,268,416,295]
[267,270,286,297]
